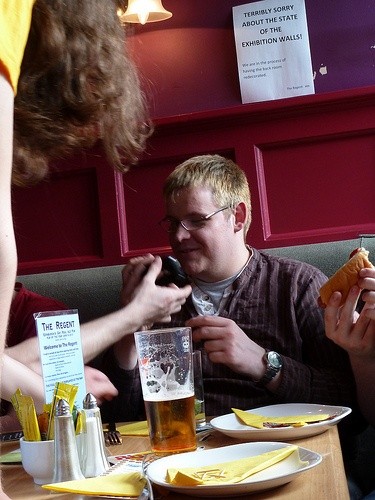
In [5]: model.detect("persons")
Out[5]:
[326,266,375,423]
[0,0,153,500]
[0,281,193,435]
[117,153,358,418]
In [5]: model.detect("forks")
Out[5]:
[104,430,123,446]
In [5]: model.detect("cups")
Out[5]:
[20,436,54,485]
[134,327,198,456]
[191,349,207,430]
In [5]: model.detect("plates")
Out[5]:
[210,403,352,442]
[146,441,323,495]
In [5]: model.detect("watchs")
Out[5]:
[252,347,284,386]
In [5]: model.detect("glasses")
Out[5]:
[158,204,230,233]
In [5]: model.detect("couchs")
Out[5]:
[16,235,375,321]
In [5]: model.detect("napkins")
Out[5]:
[166,445,298,486]
[41,471,146,497]
[104,420,149,436]
[232,407,330,428]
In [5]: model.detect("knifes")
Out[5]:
[107,416,121,445]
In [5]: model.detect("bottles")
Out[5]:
[51,399,85,494]
[76,392,110,477]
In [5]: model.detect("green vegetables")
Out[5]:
[195,402,201,415]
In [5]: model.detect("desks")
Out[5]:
[0,416,351,500]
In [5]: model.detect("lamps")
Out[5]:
[121,0,173,25]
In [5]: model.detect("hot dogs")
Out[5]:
[317,248,375,308]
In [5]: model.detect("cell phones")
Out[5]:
[0,430,23,446]
[139,255,189,289]
